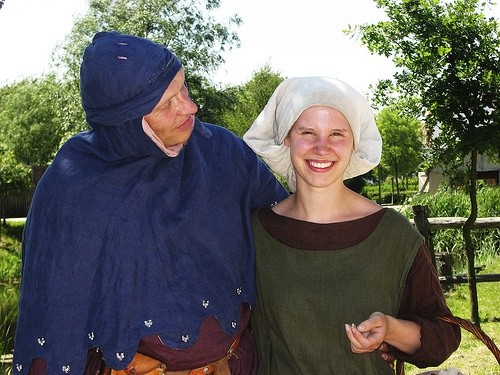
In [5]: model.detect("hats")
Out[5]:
[242,74,384,195]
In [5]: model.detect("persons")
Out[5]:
[243,76,463,375]
[13,30,292,375]
